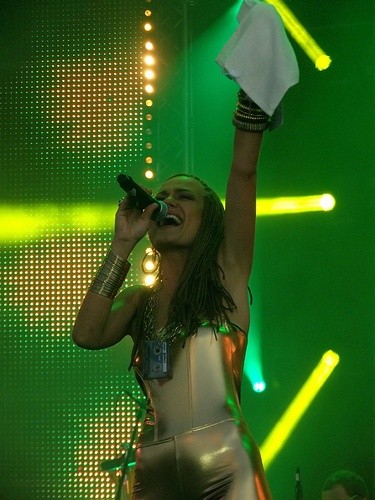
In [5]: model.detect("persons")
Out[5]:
[320,468,375,500]
[69,0,278,500]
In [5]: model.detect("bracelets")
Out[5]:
[231,87,273,132]
[88,247,130,301]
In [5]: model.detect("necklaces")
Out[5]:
[142,274,186,347]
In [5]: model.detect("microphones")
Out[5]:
[117,174,168,222]
[295,468,303,500]
